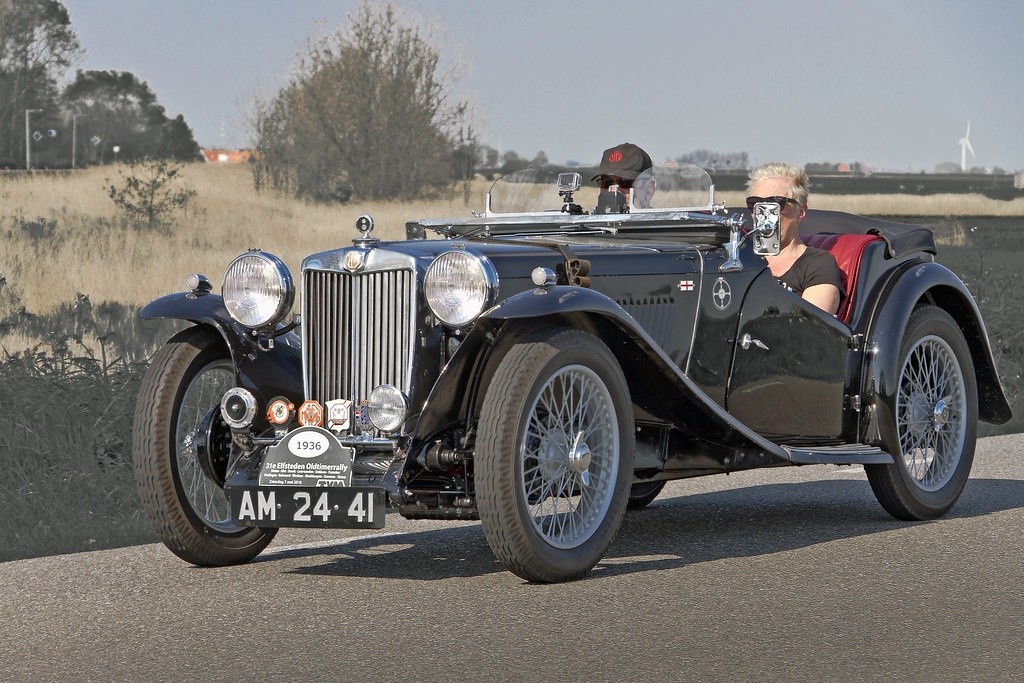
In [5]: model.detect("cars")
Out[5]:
[130,159,1014,585]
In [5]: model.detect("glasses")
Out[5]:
[746,195,798,214]
[595,178,635,189]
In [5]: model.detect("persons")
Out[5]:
[744,161,849,316]
[589,142,657,215]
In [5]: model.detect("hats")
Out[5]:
[590,143,653,182]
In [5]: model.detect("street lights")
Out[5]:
[24,108,44,169]
[71,114,87,169]
